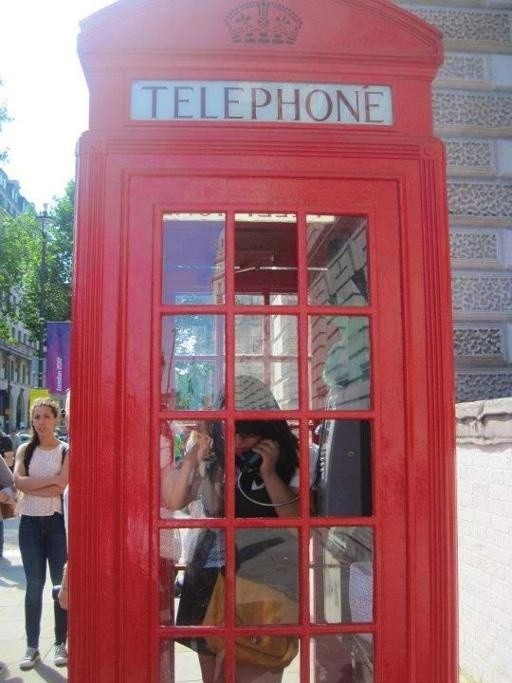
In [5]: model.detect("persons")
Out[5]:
[58,559,69,610]
[1,430,15,467]
[1,454,19,558]
[175,374,320,682]
[13,398,70,668]
[158,402,198,683]
[10,426,22,451]
[169,419,204,471]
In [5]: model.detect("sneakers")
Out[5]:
[53,642,68,666]
[19,646,41,671]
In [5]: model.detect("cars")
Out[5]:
[12,429,32,447]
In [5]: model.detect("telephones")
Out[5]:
[241,452,263,477]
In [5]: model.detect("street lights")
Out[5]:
[27,200,63,387]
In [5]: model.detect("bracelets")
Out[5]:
[59,585,68,590]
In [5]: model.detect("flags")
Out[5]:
[45,321,71,394]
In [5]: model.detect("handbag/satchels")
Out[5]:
[0,485,19,520]
[199,562,308,674]
